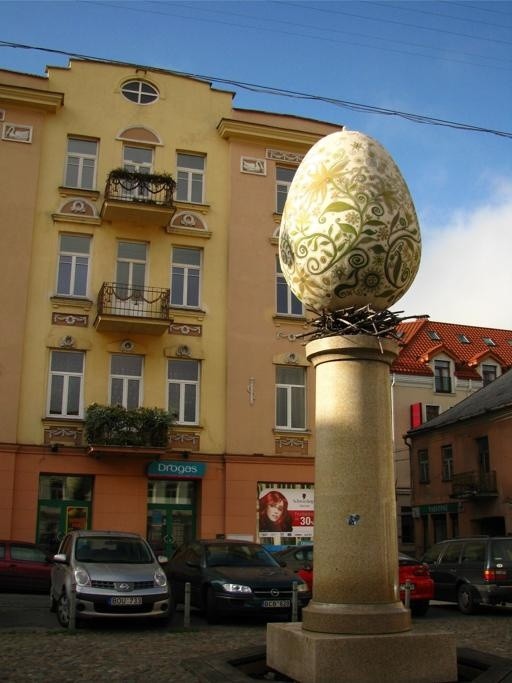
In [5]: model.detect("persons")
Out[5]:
[259,490,293,533]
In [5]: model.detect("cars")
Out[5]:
[419,536,512,614]
[164,538,310,625]
[0,539,54,592]
[269,543,435,616]
[49,531,176,626]
